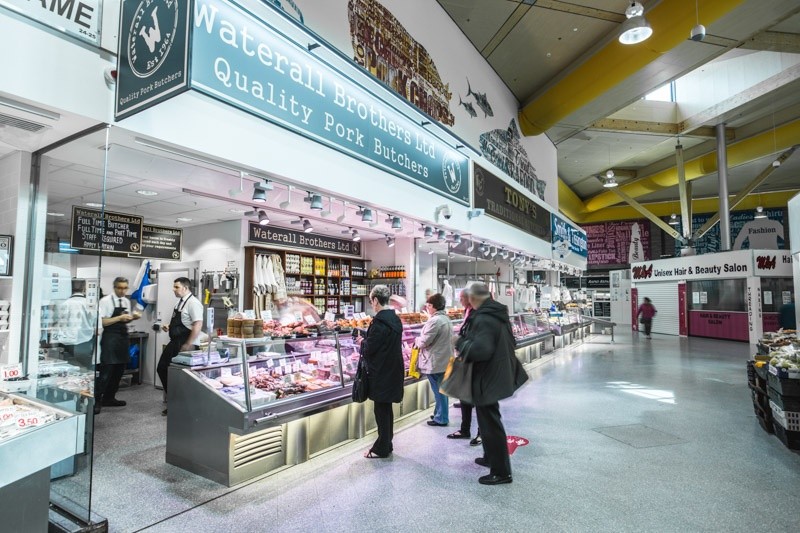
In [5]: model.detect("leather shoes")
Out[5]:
[479,474,512,485]
[475,457,491,468]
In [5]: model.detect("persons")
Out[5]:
[56,278,103,415]
[635,297,655,340]
[99,276,142,407]
[157,276,204,417]
[355,284,406,462]
[451,280,529,485]
[414,293,455,428]
[776,300,796,330]
[447,288,485,446]
[272,288,322,326]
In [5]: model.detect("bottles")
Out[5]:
[284,254,406,315]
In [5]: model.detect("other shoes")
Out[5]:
[364,450,388,458]
[453,402,474,409]
[446,430,471,439]
[470,434,483,446]
[646,335,651,339]
[426,415,447,426]
[161,409,168,416]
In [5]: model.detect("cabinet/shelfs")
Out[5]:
[243,242,370,319]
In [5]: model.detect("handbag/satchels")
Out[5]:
[640,318,646,323]
[439,357,458,398]
[437,341,476,404]
[408,343,422,379]
[352,341,371,402]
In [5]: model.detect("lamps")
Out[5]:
[754,158,767,218]
[617,0,654,45]
[603,140,620,188]
[244,174,589,279]
[668,185,680,224]
[689,0,706,41]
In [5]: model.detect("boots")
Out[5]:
[94,377,105,414]
[101,380,126,406]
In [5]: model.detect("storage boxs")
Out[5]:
[163,301,599,488]
[745,343,800,451]
